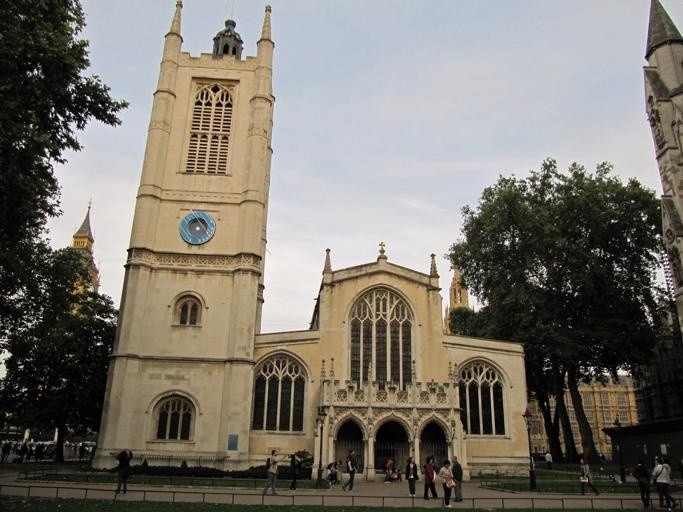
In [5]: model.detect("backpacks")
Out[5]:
[266,458,274,470]
[328,463,334,469]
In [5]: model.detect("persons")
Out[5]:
[0,439,85,461]
[115,448,134,494]
[262,449,287,495]
[341,449,359,491]
[438,460,454,508]
[289,454,298,490]
[422,456,438,500]
[451,455,463,502]
[327,460,342,485]
[631,456,675,508]
[384,458,401,484]
[579,457,600,496]
[545,450,553,470]
[405,456,419,496]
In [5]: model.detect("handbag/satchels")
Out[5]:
[654,479,657,485]
[638,477,649,488]
[580,476,589,483]
[446,478,456,488]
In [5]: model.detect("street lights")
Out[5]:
[315,407,327,488]
[521,406,536,492]
[612,413,625,483]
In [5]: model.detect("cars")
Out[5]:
[1,438,97,459]
[531,452,611,463]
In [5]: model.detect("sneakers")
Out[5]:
[444,504,454,508]
[409,494,415,497]
[342,485,347,491]
[452,496,463,502]
